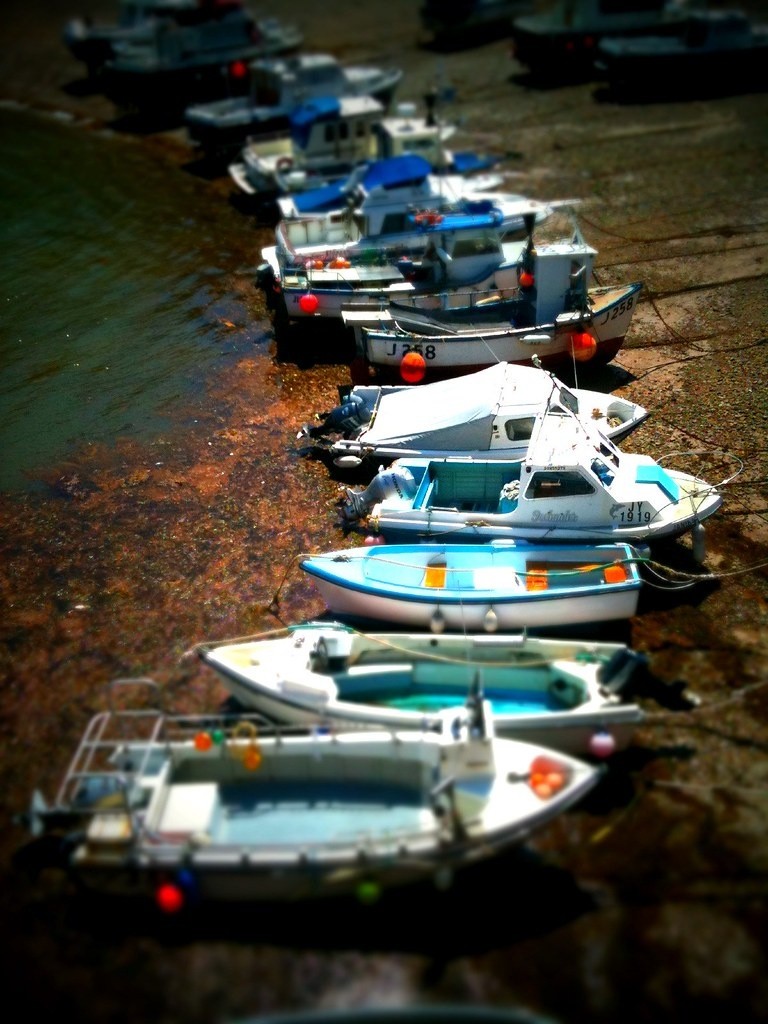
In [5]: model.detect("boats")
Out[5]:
[17,707,614,913]
[345,230,645,388]
[295,540,646,634]
[329,412,726,558]
[62,0,768,327]
[193,621,701,761]
[298,360,653,484]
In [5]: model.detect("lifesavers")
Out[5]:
[415,214,443,224]
[277,157,293,170]
